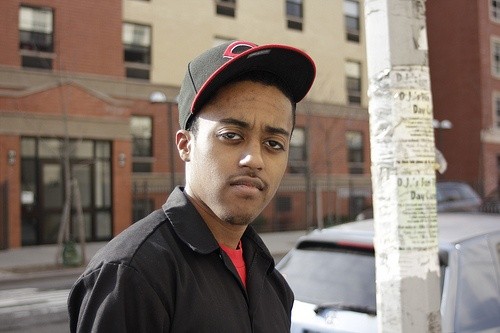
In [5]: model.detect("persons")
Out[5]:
[67,40,317,333]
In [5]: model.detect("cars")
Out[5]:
[435,181,484,214]
[274,212,500,333]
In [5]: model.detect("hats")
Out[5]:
[178,41,316,129]
